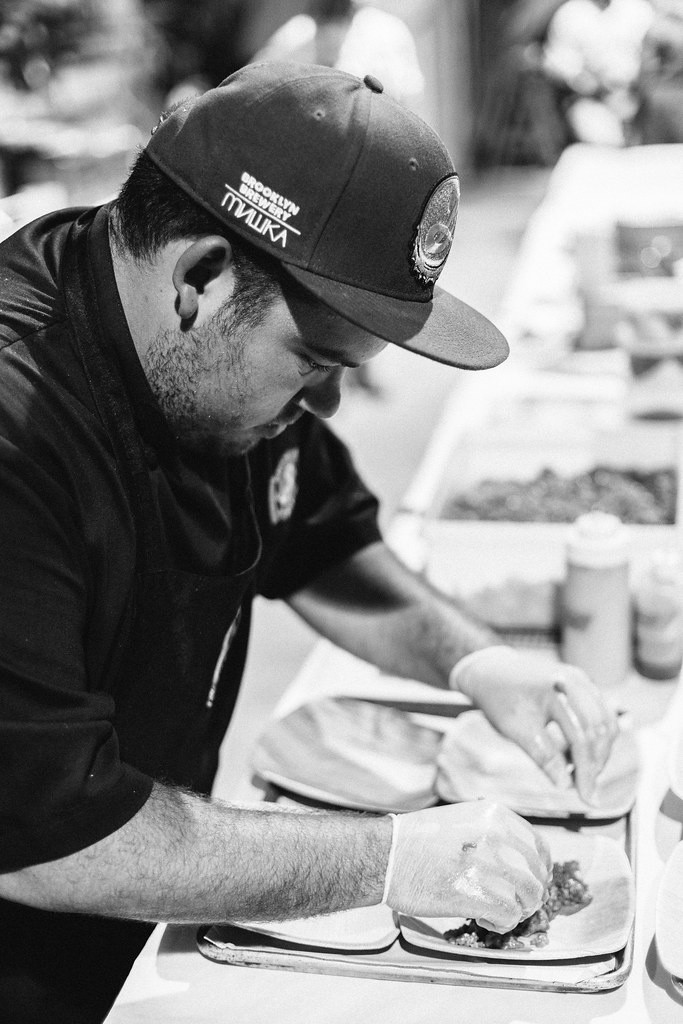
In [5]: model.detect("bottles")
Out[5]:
[633,569,683,679]
[560,561,632,687]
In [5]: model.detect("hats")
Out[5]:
[145,62,510,371]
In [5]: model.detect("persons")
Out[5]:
[0,62,618,1024]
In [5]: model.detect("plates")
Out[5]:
[250,698,455,813]
[654,685,683,1001]
[400,830,633,960]
[227,904,398,951]
[436,710,642,820]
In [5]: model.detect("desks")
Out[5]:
[96,140,683,1024]
